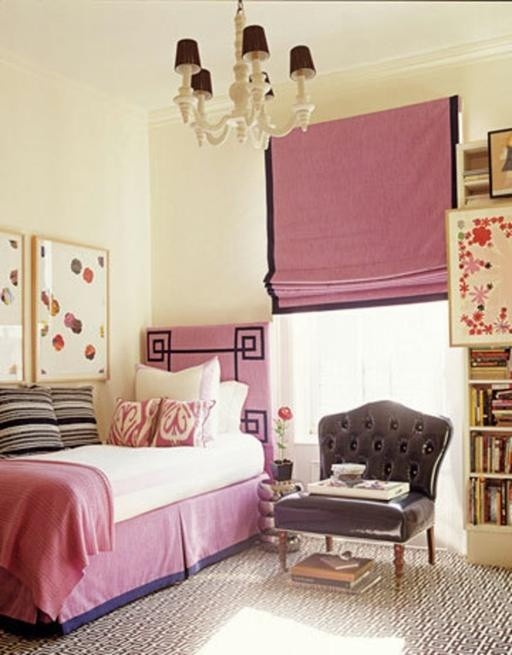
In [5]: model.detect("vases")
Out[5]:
[271,460,294,484]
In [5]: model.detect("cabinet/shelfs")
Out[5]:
[456,139,512,570]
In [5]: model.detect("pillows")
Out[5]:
[0,385,63,455]
[135,357,220,401]
[107,396,162,448]
[209,380,248,435]
[150,398,215,448]
[20,384,104,449]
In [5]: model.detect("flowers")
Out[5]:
[273,405,293,461]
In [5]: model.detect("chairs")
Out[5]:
[273,401,452,596]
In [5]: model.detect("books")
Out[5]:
[467,345,512,530]
[464,167,510,205]
[291,552,381,595]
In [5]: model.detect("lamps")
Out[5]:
[170,0,314,151]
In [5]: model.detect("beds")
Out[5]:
[0,318,294,639]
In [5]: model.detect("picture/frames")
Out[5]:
[31,234,111,384]
[487,128,512,198]
[443,202,512,348]
[0,228,28,386]
